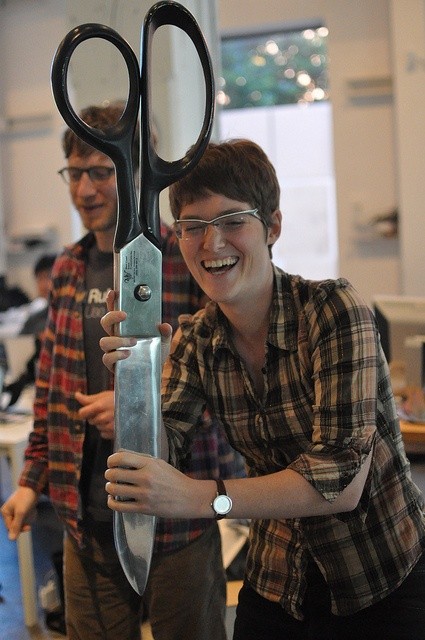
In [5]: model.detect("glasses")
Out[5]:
[174,207,268,240]
[59,166,114,186]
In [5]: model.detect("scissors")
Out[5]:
[51,0,215,597]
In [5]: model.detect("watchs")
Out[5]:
[210,478,233,520]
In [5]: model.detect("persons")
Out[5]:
[0,100,228,640]
[34,255,59,299]
[99,137,423,640]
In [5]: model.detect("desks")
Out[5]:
[1,370,40,627]
